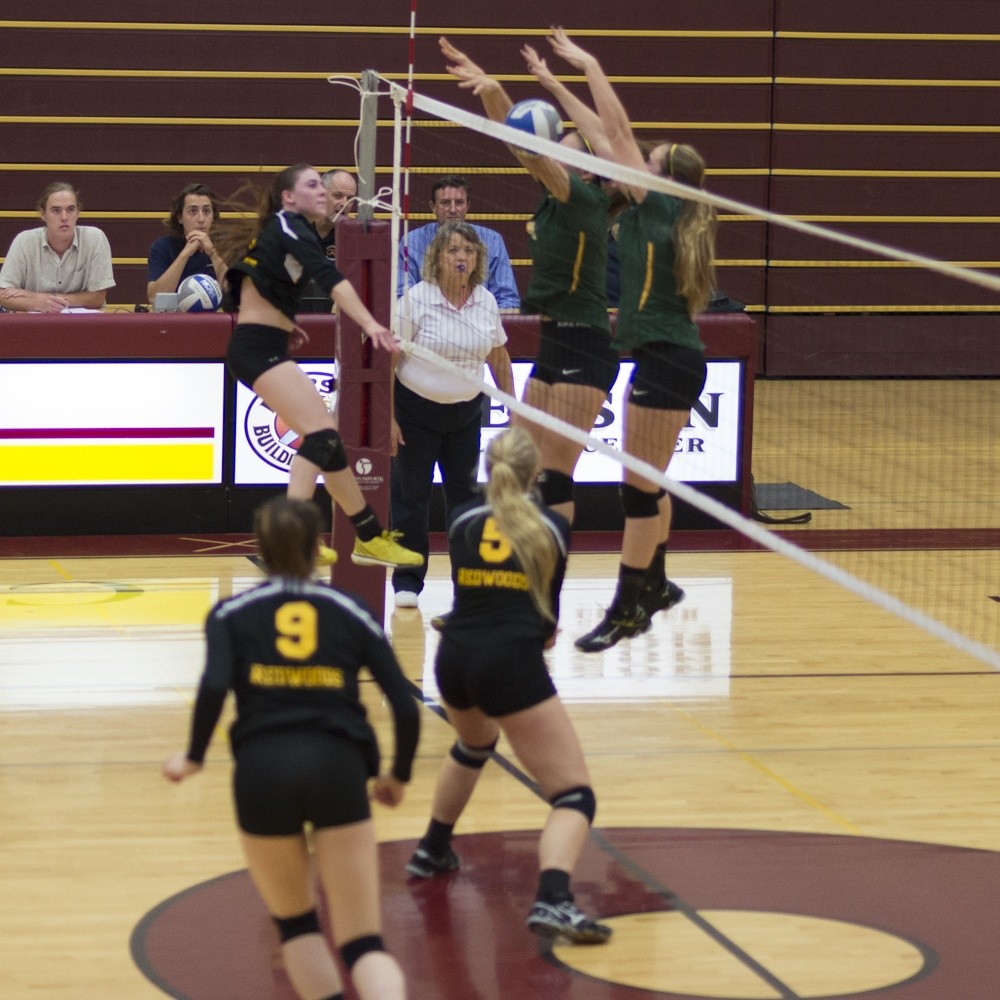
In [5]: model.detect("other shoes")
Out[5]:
[394,591,419,607]
[432,611,450,629]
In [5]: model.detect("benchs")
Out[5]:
[0,0,1000,378]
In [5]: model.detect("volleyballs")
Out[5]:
[178,273,223,313]
[504,100,563,159]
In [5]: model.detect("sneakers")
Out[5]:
[351,529,425,568]
[528,898,612,943]
[314,546,337,566]
[405,840,457,880]
[574,604,651,652]
[640,581,686,617]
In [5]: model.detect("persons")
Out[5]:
[161,495,420,1000]
[204,163,425,567]
[392,25,715,655]
[0,183,116,314]
[146,183,239,304]
[404,428,619,943]
